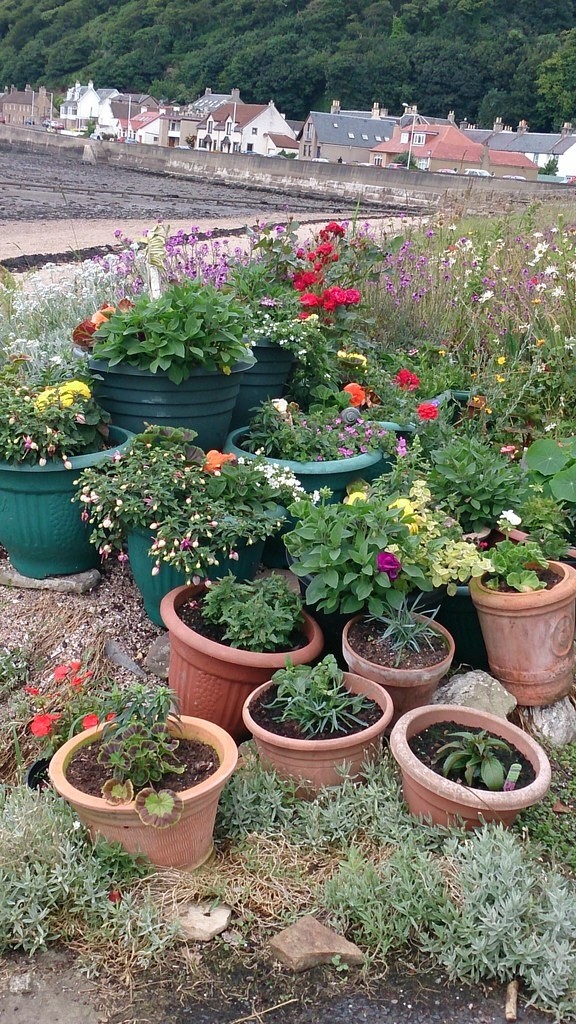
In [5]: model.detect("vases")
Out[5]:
[444,390,477,427]
[222,426,399,508]
[88,352,256,454]
[0,424,138,579]
[475,559,576,708]
[227,335,294,433]
[456,395,486,429]
[26,757,53,796]
[363,420,415,449]
[122,504,290,631]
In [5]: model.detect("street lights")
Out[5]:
[223,98,237,154]
[24,88,35,122]
[42,90,53,123]
[119,95,132,140]
[400,101,418,170]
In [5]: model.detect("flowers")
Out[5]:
[0,349,112,470]
[72,273,260,385]
[249,394,410,482]
[69,420,323,585]
[23,657,123,791]
[470,508,573,595]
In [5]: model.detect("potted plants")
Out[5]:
[342,590,456,744]
[486,434,576,570]
[242,652,392,806]
[46,662,238,872]
[389,704,551,834]
[469,516,576,707]
[160,568,325,749]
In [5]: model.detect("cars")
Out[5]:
[173,143,576,185]
[0,116,141,145]
